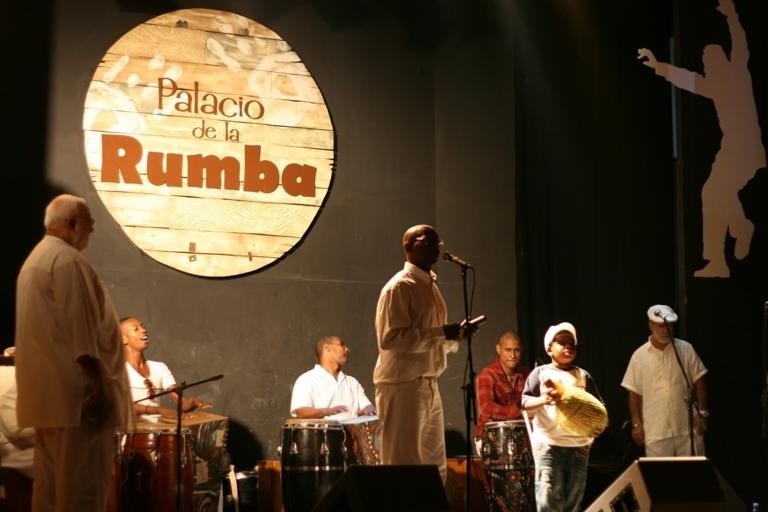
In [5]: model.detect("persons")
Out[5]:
[521,321,607,512]
[16,192,137,511]
[373,224,478,487]
[620,304,710,458]
[115,315,212,458]
[474,329,532,458]
[637,1,767,280]
[289,334,377,419]
[0,347,38,479]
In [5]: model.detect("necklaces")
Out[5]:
[501,366,517,382]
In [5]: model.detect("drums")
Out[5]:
[123,426,194,509]
[143,412,227,496]
[481,420,532,508]
[281,418,346,512]
[328,414,381,466]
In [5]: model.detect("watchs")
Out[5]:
[632,421,642,428]
[698,409,710,417]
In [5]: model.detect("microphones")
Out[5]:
[654,309,670,321]
[442,250,472,270]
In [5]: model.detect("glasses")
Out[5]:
[410,235,445,248]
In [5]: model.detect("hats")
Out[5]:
[542,322,582,357]
[646,303,678,326]
